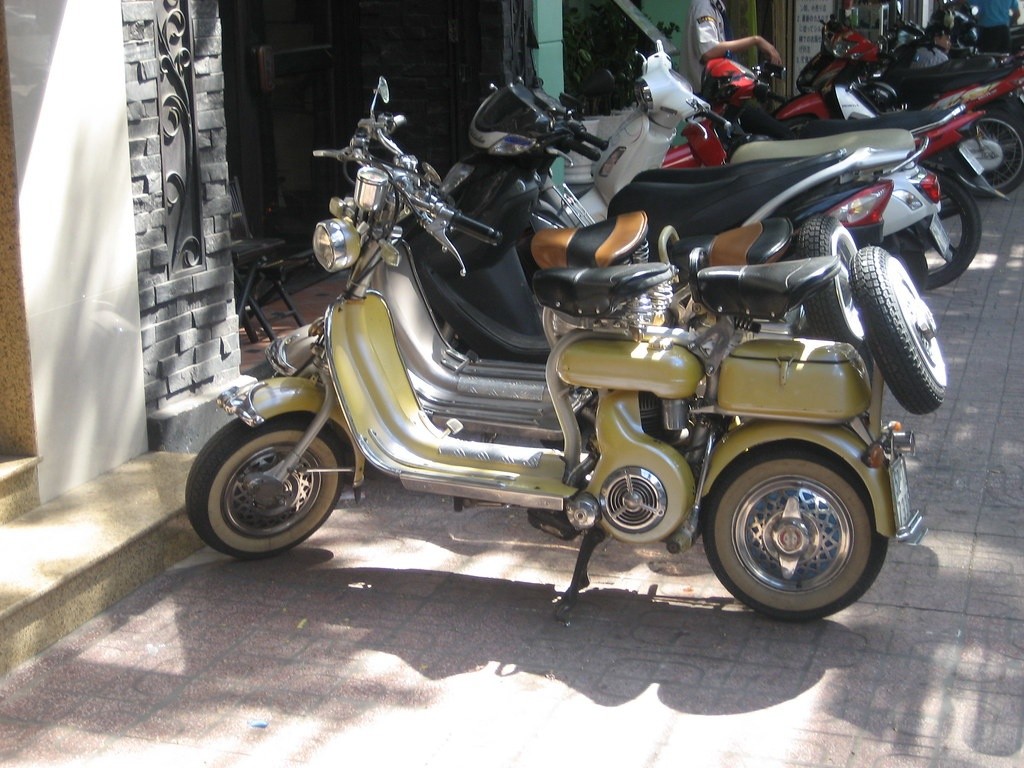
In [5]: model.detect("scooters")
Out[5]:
[264,2,1024,491]
[185,76,948,626]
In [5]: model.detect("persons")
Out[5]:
[957,0,1021,52]
[678,0,783,94]
[911,21,952,70]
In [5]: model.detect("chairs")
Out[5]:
[228,174,308,343]
